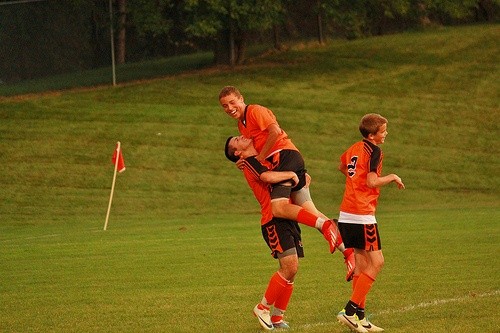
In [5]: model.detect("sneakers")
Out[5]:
[344,252,356,282]
[324,219,338,254]
[273,319,290,329]
[336,308,369,333]
[253,301,275,331]
[359,317,384,332]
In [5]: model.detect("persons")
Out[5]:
[219,86,356,281]
[225,135,312,331]
[337,113,406,332]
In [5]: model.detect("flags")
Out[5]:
[112,141,126,172]
